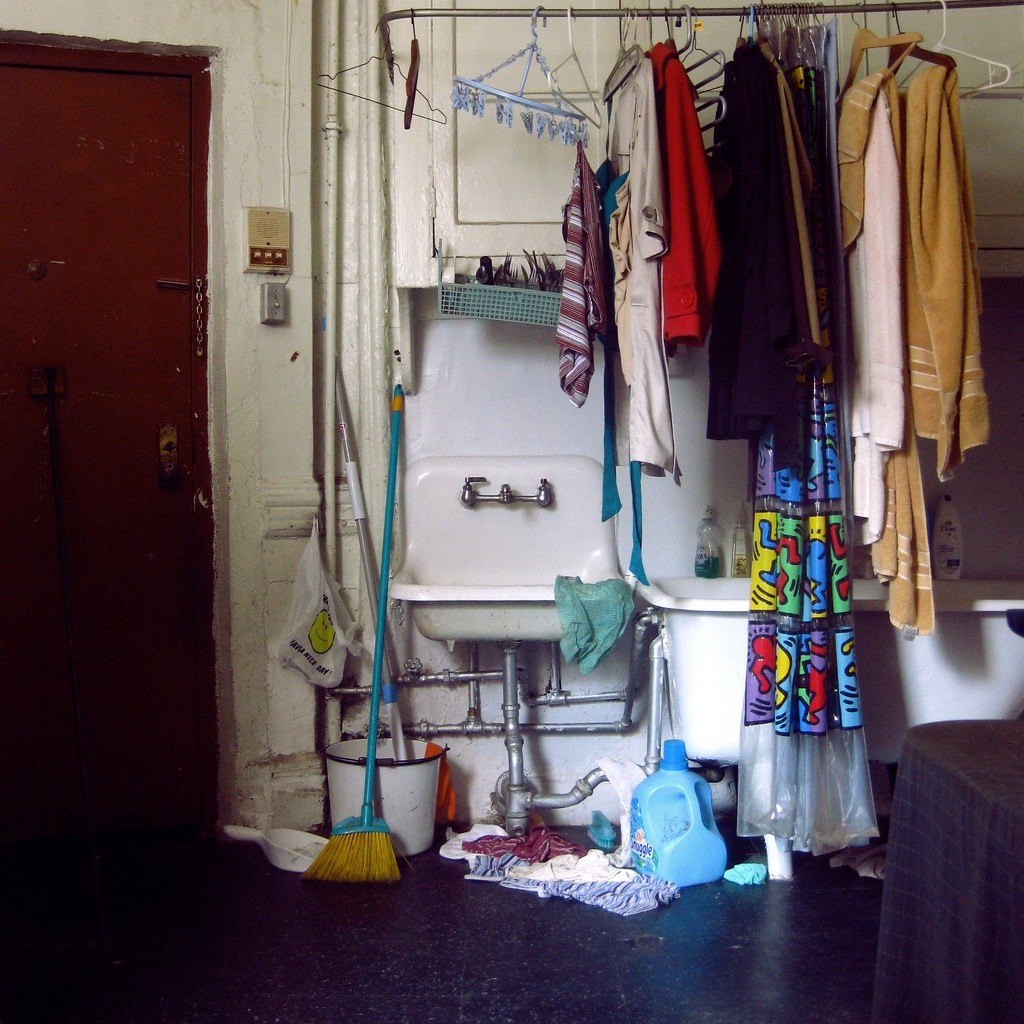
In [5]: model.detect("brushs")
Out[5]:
[587,810,617,850]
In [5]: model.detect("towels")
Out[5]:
[553,139,610,409]
[837,66,992,638]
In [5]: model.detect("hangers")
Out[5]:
[316,0,1012,148]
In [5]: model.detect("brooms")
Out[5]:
[297,384,414,884]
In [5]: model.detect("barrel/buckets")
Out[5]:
[324,737,444,855]
[630,739,726,886]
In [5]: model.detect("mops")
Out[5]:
[333,365,409,763]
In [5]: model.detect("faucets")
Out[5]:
[461,475,487,504]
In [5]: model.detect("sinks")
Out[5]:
[387,569,636,644]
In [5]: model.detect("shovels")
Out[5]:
[221,822,331,872]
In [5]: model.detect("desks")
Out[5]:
[875,718,1024,1024]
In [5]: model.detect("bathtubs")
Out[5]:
[634,575,1024,762]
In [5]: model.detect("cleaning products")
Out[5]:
[694,499,751,578]
[628,739,727,887]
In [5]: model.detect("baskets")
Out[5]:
[436,239,560,327]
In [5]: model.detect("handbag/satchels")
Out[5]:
[278,518,364,688]
